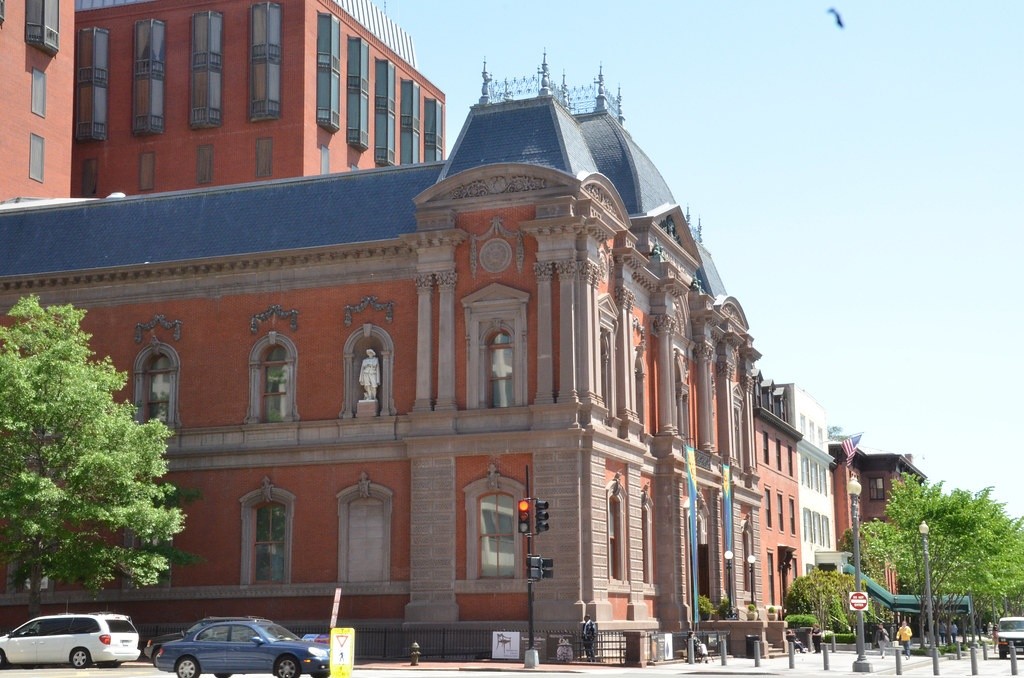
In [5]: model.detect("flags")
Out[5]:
[841,435,862,465]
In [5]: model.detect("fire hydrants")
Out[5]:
[409,641,421,666]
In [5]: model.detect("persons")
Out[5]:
[359,349,380,400]
[812,624,821,653]
[687,632,701,662]
[993,624,997,653]
[896,621,912,660]
[787,630,806,653]
[875,623,889,659]
[582,614,596,661]
[940,622,958,643]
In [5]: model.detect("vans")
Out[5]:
[997,617,1024,659]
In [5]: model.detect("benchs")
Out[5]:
[684,637,715,663]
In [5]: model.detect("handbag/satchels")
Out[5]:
[884,636,890,644]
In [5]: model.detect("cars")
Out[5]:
[144,616,330,678]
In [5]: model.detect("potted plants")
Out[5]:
[698,594,717,620]
[717,596,731,620]
[767,606,778,621]
[746,603,758,620]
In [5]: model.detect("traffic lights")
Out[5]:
[518,500,530,534]
[536,500,549,535]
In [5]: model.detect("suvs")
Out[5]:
[0,612,141,669]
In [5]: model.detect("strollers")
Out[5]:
[695,640,714,664]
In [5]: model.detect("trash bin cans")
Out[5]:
[301,634,320,643]
[314,634,330,645]
[745,634,759,659]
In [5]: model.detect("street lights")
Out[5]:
[918,520,939,675]
[748,555,756,604]
[724,551,734,620]
[846,475,871,672]
